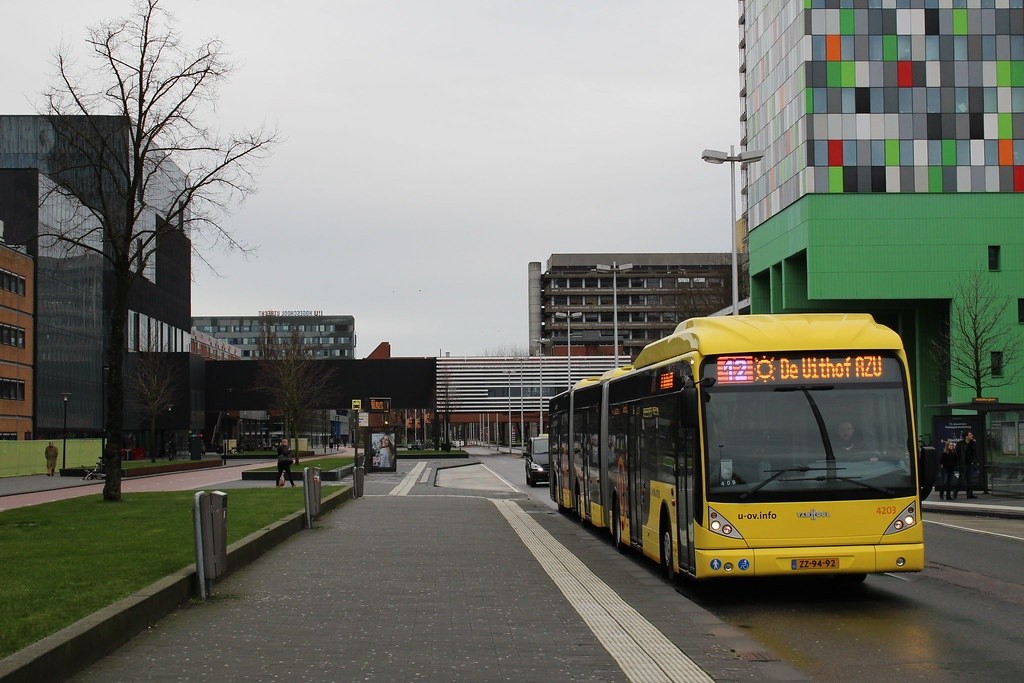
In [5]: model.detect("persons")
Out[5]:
[833,420,877,460]
[956,431,980,499]
[276,439,296,486]
[940,442,960,500]
[373,434,394,467]
[45,441,58,476]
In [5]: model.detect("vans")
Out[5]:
[522,434,550,487]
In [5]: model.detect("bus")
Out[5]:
[545,313,926,599]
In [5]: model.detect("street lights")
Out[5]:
[594,260,633,369]
[530,338,550,436]
[503,368,517,456]
[514,355,530,457]
[555,312,582,390]
[167,403,174,441]
[701,144,766,313]
[61,392,72,469]
